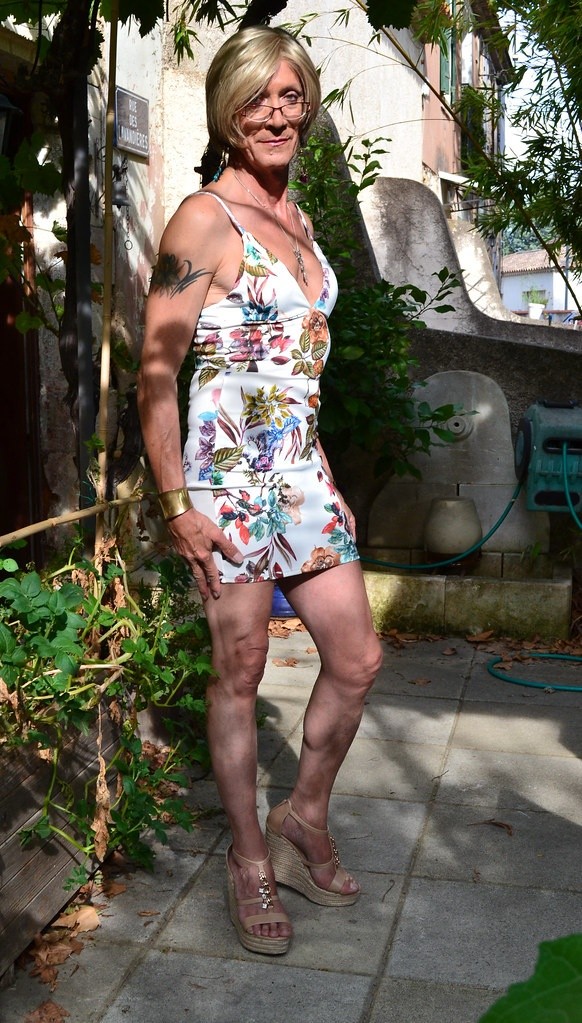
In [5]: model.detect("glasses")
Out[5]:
[242,101,311,122]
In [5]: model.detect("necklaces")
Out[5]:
[228,165,308,287]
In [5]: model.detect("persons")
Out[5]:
[137,25,384,954]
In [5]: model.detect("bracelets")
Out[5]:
[156,487,193,521]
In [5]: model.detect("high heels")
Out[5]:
[225,842,292,955]
[267,799,361,907]
